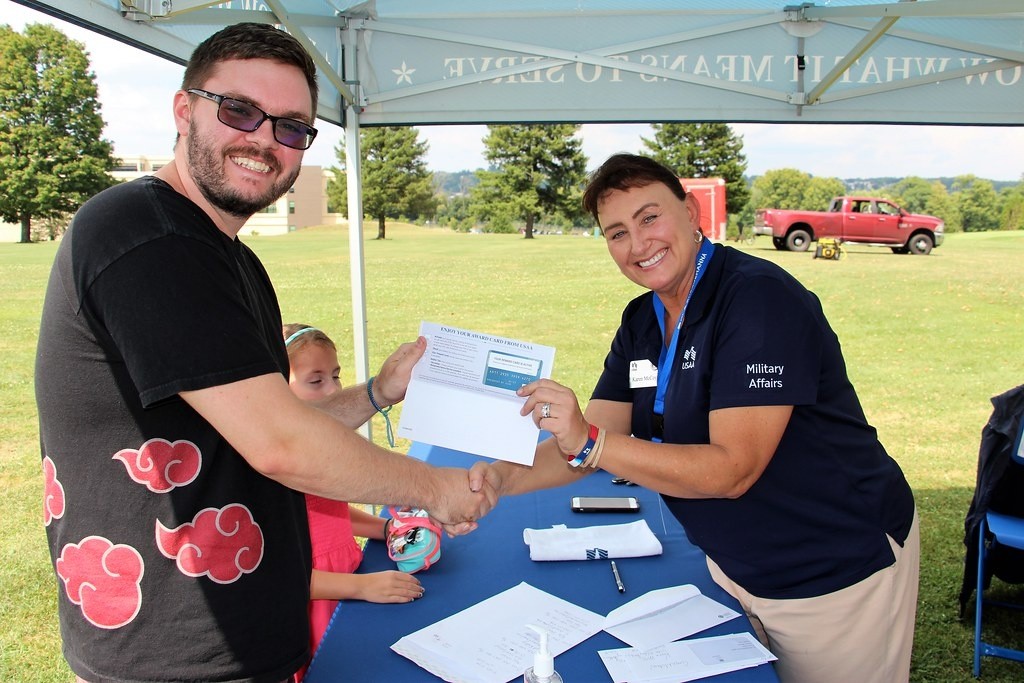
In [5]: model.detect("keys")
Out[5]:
[611,477,635,487]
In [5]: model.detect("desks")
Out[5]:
[298,430,781,683]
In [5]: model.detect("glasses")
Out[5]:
[186,88,318,151]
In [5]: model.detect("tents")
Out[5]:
[0,0,1024,516]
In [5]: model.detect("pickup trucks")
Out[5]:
[751,195,946,256]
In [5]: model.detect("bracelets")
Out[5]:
[567,424,607,469]
[367,376,395,448]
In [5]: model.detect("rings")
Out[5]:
[542,403,550,418]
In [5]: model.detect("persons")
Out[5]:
[280,323,425,655]
[33,20,496,683]
[429,154,921,683]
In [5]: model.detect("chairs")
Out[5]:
[956,383,1024,676]
